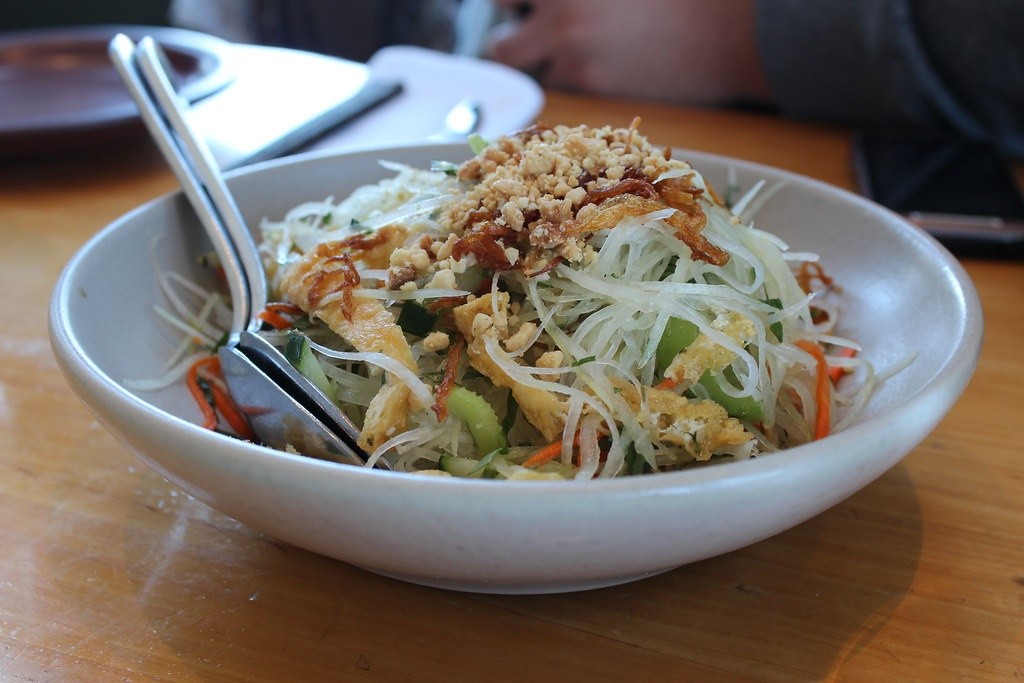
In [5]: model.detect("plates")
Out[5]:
[0,27,237,162]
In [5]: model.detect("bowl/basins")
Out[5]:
[45,140,986,597]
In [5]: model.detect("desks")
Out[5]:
[0,92,1024,683]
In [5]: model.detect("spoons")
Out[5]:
[106,31,392,467]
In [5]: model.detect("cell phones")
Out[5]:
[848,125,1024,264]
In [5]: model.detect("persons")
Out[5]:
[481,0,1024,150]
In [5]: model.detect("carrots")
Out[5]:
[186,302,858,466]
